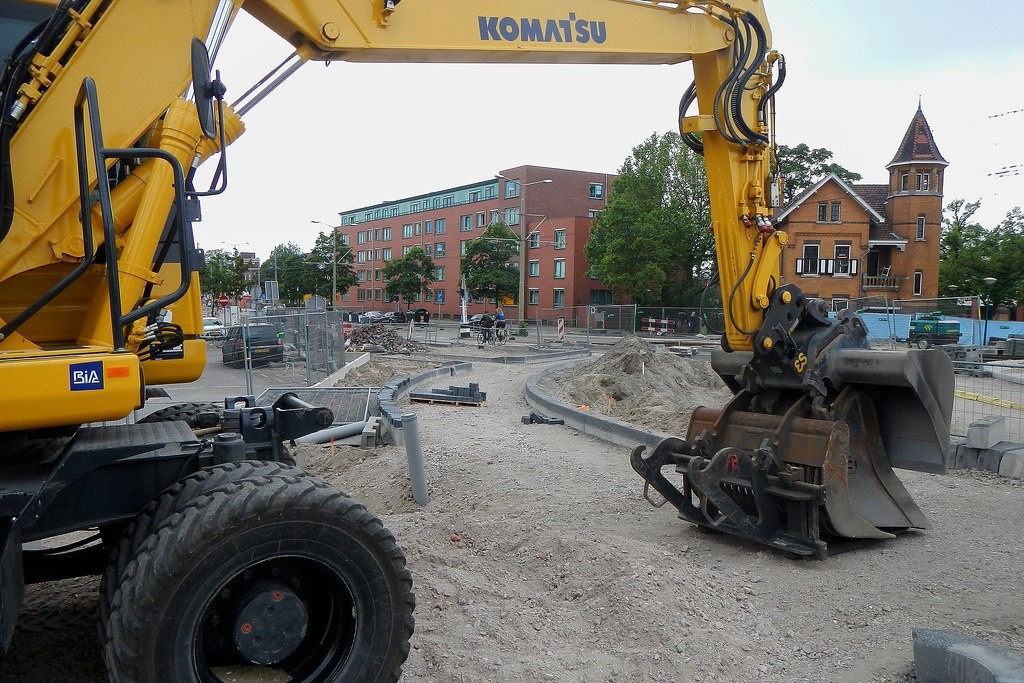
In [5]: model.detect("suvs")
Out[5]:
[222,321,283,367]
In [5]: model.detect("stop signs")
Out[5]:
[219,296,228,307]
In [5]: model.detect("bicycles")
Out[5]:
[492,324,508,346]
[475,323,496,346]
[200,331,224,346]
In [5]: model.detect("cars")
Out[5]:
[360,311,382,324]
[202,317,225,338]
[469,314,496,327]
[379,312,405,324]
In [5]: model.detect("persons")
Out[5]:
[479,310,494,343]
[493,308,509,341]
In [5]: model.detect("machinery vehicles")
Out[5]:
[0,1,957,683]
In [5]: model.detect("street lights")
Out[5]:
[220,241,251,267]
[311,220,358,312]
[946,277,997,347]
[494,173,553,320]
